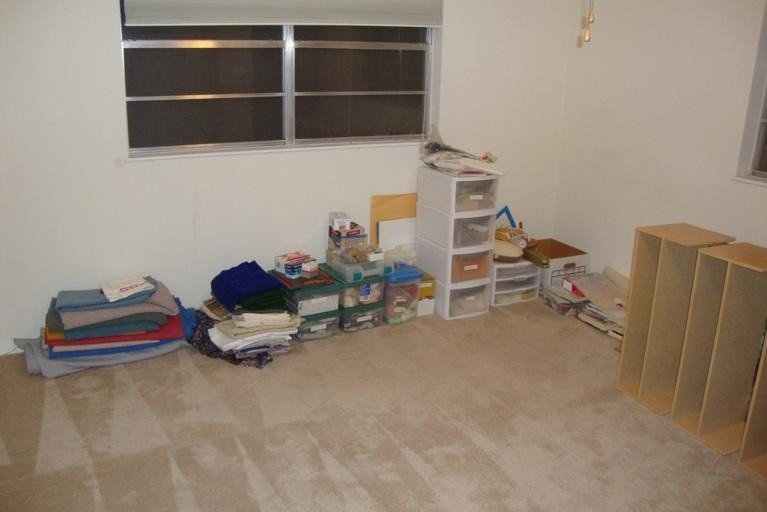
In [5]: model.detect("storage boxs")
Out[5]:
[268,226,436,343]
[526,238,590,298]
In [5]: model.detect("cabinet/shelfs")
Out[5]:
[613,222,766,478]
[415,166,499,320]
[491,258,541,307]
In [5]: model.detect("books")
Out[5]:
[545,286,625,340]
